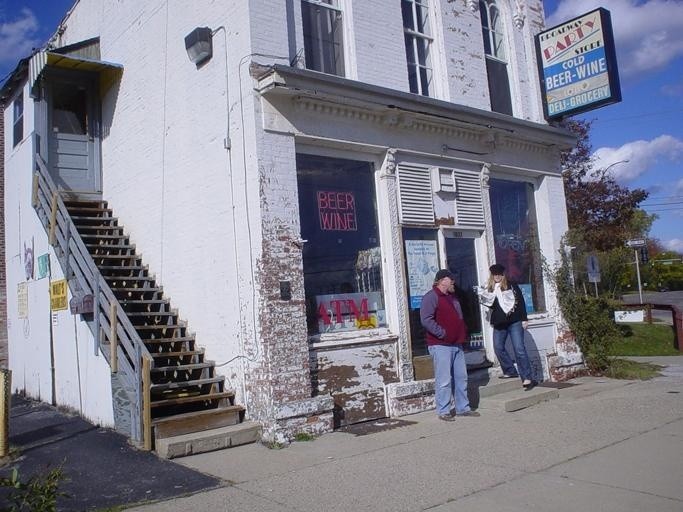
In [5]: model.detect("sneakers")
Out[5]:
[456,410,481,417]
[439,414,455,421]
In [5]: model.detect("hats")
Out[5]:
[489,264,506,275]
[434,269,457,281]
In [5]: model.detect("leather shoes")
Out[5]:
[523,380,534,388]
[498,375,518,379]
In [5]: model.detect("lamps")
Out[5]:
[183,27,213,68]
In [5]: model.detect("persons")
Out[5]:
[482,264,534,386]
[419,268,480,421]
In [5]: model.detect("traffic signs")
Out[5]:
[624,239,646,248]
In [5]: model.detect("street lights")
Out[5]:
[602,160,630,180]
